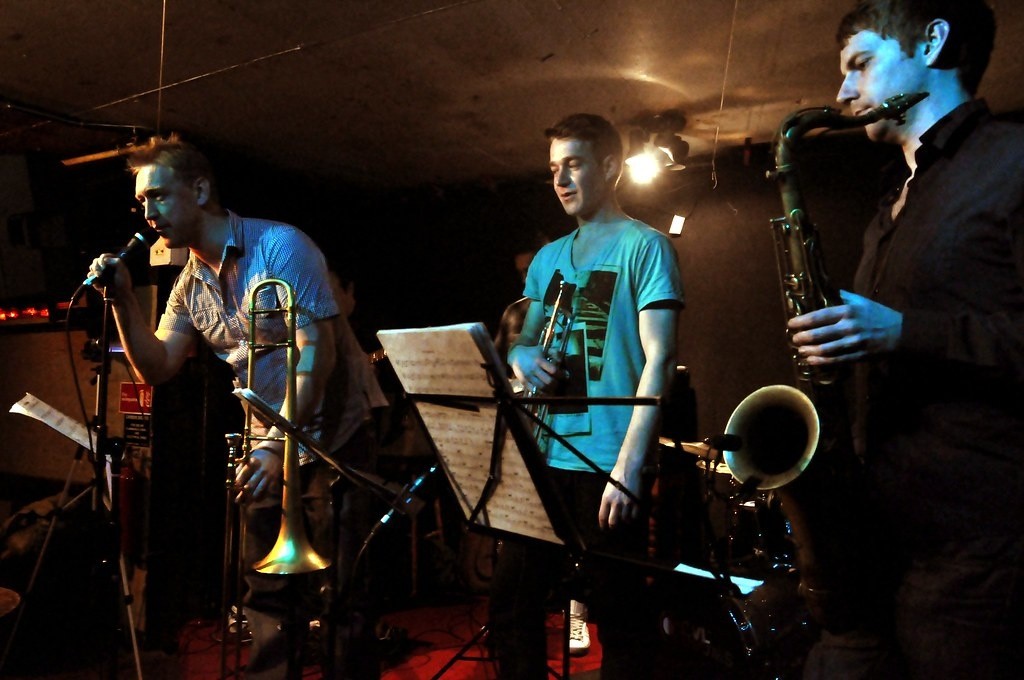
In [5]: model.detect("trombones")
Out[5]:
[218,276,333,680]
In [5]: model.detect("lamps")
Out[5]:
[622,129,652,165]
[657,125,694,164]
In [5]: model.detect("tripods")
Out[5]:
[0,275,145,679]
[433,541,561,680]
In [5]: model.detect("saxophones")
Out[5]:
[714,86,934,640]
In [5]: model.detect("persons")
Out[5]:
[476,108,684,680]
[84,135,395,680]
[777,0,1024,680]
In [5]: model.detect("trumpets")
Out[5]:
[499,279,578,444]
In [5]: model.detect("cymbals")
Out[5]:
[655,435,726,464]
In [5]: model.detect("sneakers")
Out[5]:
[567,612,591,655]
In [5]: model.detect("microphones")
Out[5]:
[82,226,161,289]
